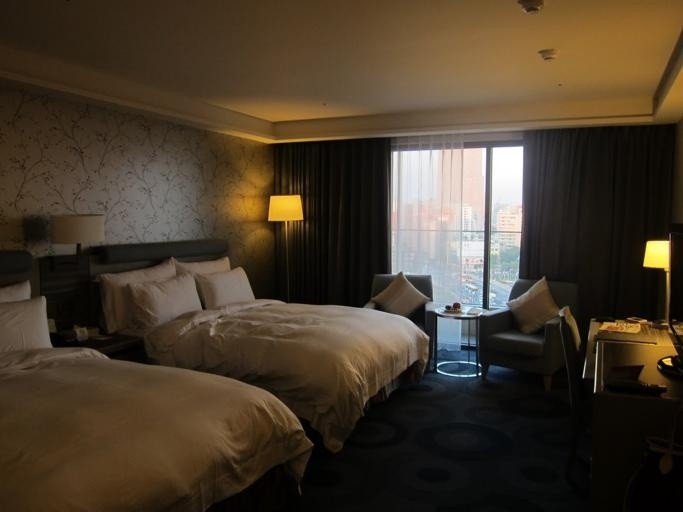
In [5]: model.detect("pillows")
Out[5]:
[0,295,55,352]
[505,276,560,334]
[371,270,431,318]
[174,255,231,277]
[127,271,203,329]
[194,266,256,310]
[0,280,32,302]
[96,256,177,335]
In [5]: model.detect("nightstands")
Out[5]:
[39,255,149,365]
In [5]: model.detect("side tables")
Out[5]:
[434,306,489,377]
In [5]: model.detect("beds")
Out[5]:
[0,247,314,512]
[81,238,430,452]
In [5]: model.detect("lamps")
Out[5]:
[642,232,672,327]
[267,194,304,301]
[50,213,105,264]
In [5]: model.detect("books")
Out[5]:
[599,322,641,334]
[594,327,658,343]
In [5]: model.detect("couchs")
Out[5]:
[363,273,435,372]
[478,279,579,394]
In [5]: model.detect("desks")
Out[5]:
[588,318,683,457]
